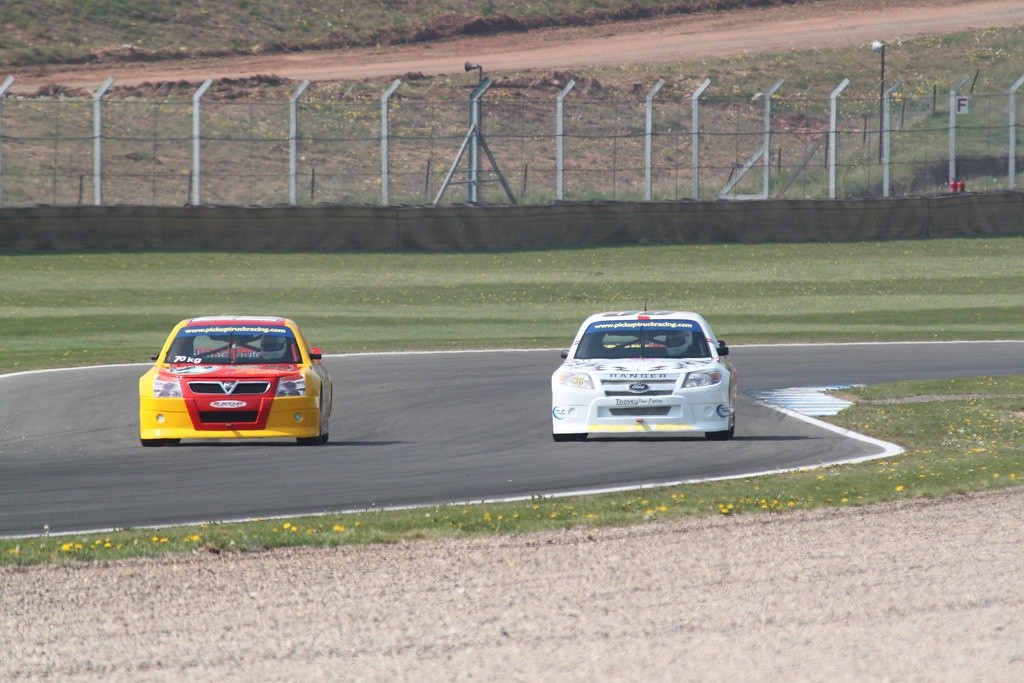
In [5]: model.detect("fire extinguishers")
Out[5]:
[948,178,958,192]
[958,178,964,192]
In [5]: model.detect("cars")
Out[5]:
[136,315,336,446]
[549,305,737,444]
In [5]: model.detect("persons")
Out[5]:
[259,335,287,362]
[664,331,693,359]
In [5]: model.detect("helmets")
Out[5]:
[665,331,693,356]
[260,335,287,360]
